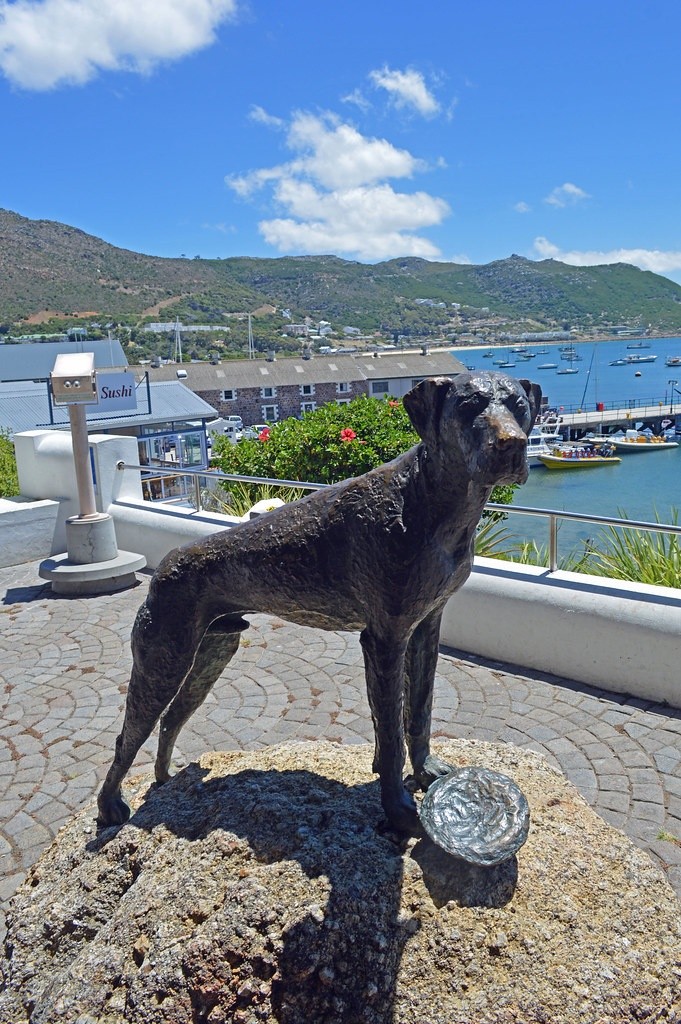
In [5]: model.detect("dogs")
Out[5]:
[96,370,542,827]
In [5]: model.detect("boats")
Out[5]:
[527,414,560,457]
[577,432,608,442]
[627,343,651,349]
[624,354,659,363]
[634,371,642,376]
[537,452,621,469]
[608,358,630,366]
[665,357,681,365]
[482,338,583,374]
[601,436,679,450]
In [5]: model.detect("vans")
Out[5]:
[224,415,243,430]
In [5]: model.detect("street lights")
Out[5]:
[668,380,678,414]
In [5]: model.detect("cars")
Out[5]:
[254,424,269,435]
